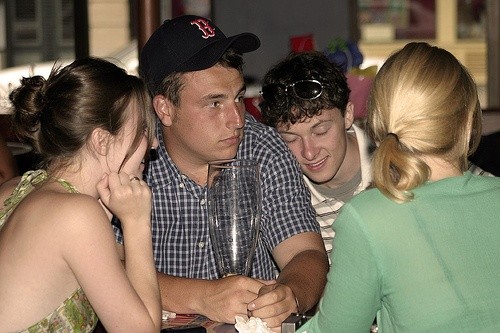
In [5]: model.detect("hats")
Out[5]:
[139,14,259,88]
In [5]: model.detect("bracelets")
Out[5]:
[292,289,303,312]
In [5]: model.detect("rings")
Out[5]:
[130,176,140,182]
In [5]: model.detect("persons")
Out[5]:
[0,58,164,333]
[292,41,500,333]
[259,53,495,269]
[109,14,329,333]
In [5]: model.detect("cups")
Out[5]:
[205,159,262,279]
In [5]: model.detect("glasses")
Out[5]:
[262,81,337,107]
